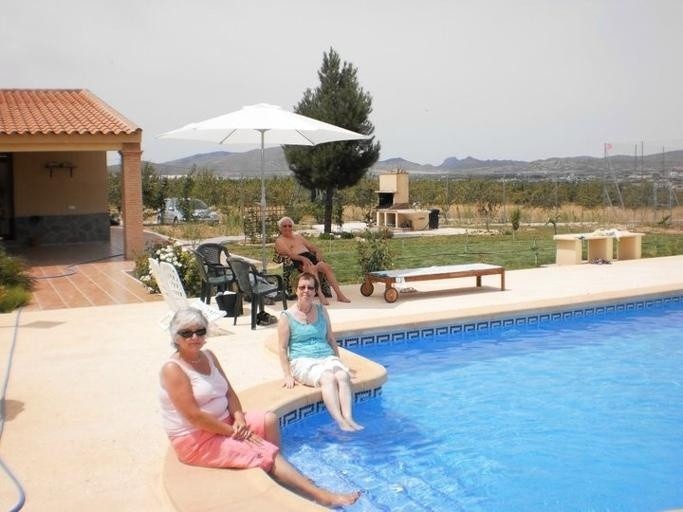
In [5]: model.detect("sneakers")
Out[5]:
[256,310,276,325]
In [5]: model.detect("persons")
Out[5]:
[156,305,362,508]
[274,272,364,435]
[273,216,352,305]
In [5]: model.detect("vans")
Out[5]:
[154,197,221,226]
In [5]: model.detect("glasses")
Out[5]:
[177,326,206,338]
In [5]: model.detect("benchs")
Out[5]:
[360,262,505,303]
[552,231,645,264]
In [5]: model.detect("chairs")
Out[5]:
[224,256,287,331]
[274,248,331,298]
[193,243,238,305]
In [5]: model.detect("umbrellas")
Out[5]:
[156,102,373,327]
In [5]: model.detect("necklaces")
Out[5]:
[293,302,315,315]
[178,348,204,365]
